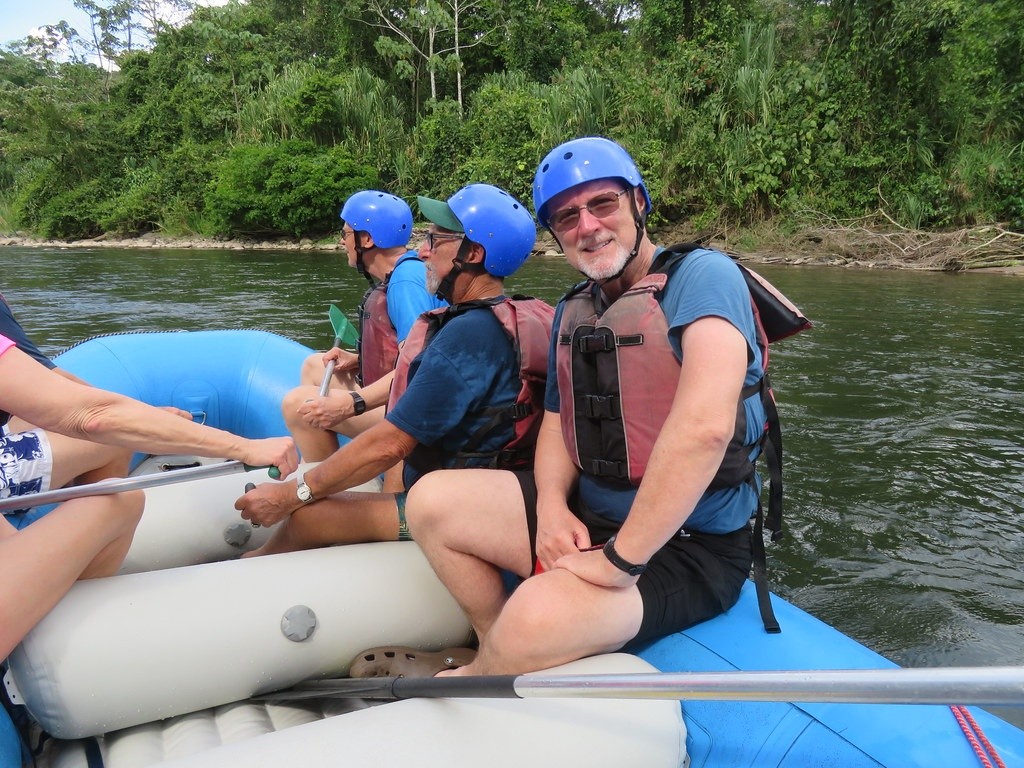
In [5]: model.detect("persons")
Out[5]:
[235,183,556,558]
[349,137,811,681]
[282,191,451,465]
[0,295,193,515]
[0,334,300,666]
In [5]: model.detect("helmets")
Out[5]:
[447,183,537,277]
[530,136,652,228]
[341,190,414,249]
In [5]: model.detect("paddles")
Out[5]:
[0,461,280,510]
[246,662,1024,704]
[308,304,361,400]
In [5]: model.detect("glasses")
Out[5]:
[425,233,462,251]
[548,190,627,233]
[340,229,353,239]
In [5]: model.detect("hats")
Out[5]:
[416,195,464,232]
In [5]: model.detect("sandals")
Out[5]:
[349,645,476,678]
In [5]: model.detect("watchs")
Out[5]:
[350,392,366,416]
[297,472,315,504]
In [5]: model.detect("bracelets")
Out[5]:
[603,536,647,575]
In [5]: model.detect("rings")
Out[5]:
[252,524,260,528]
